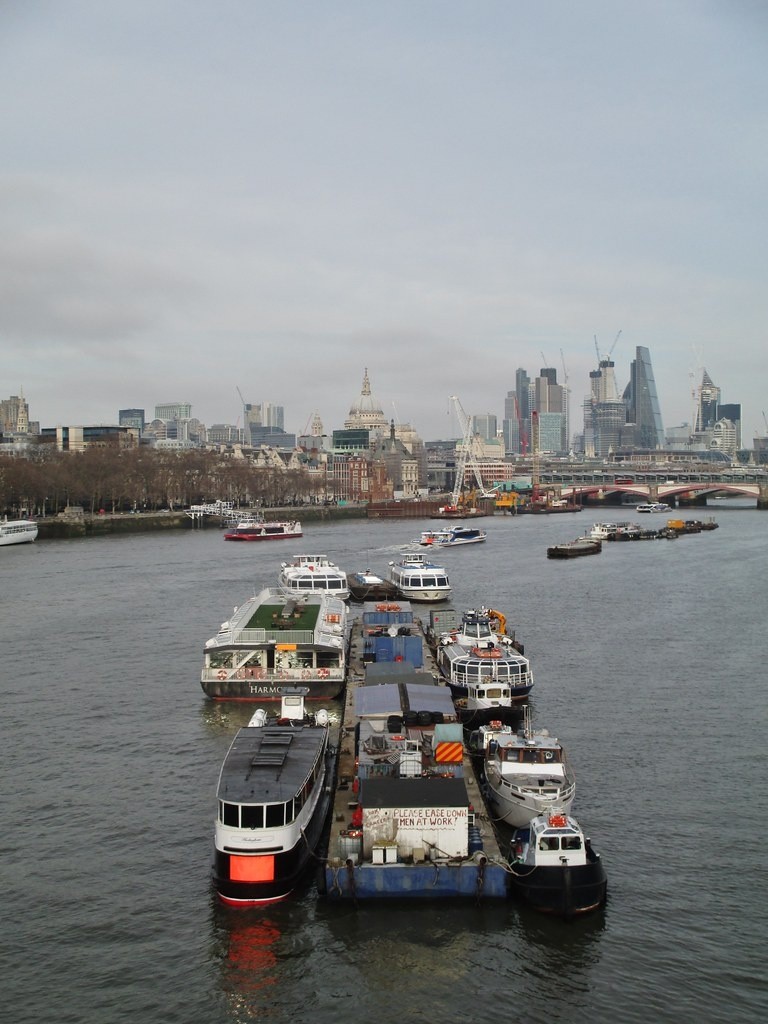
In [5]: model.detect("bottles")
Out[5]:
[510,838,523,861]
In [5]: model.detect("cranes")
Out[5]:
[536,328,623,389]
[447,394,502,501]
[438,416,475,513]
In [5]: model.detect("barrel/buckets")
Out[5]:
[468,826,483,856]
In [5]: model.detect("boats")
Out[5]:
[0,520,38,546]
[196,501,719,919]
[223,518,303,541]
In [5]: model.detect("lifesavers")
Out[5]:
[216,670,228,681]
[549,815,567,828]
[391,735,405,741]
[489,720,501,724]
[317,668,329,679]
